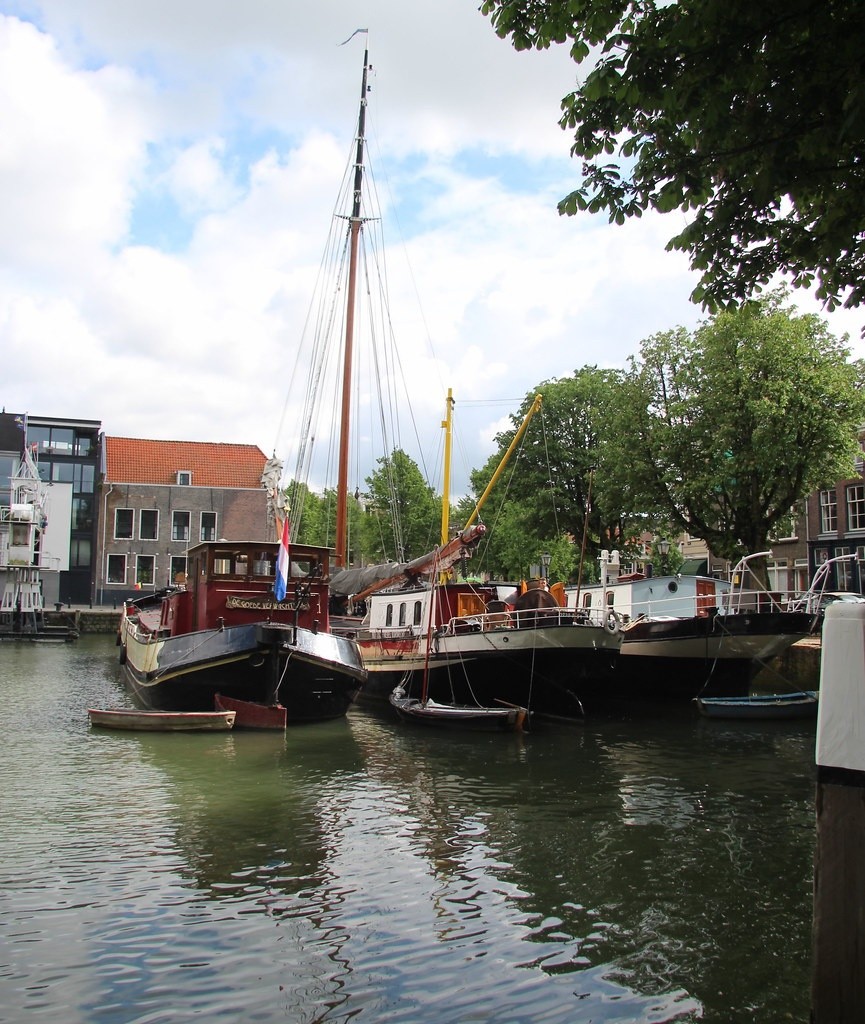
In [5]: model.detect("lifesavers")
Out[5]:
[603,609,621,635]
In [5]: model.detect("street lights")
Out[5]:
[656,536,671,576]
[541,552,552,582]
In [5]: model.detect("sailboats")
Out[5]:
[275,28,828,707]
[388,543,534,728]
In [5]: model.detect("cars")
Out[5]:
[809,592,865,616]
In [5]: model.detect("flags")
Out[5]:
[274,516,289,604]
[134,582,143,590]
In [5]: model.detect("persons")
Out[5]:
[485,576,550,631]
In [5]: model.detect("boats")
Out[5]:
[215,696,288,731]
[88,704,237,732]
[691,690,818,718]
[118,539,371,723]
